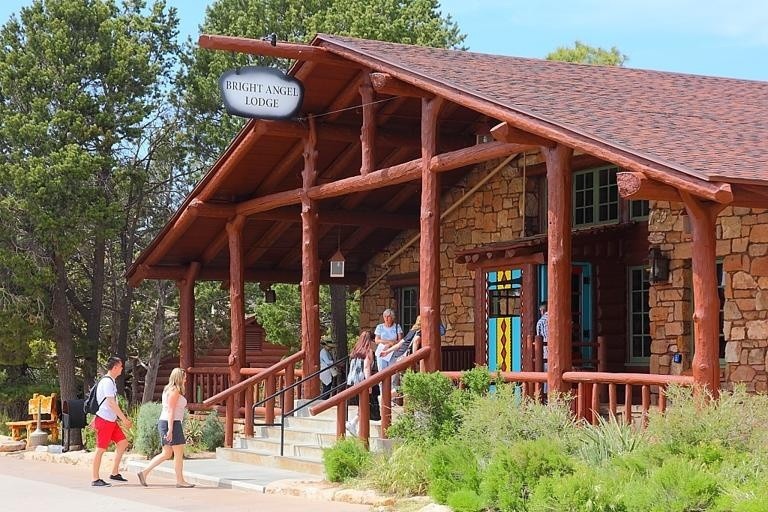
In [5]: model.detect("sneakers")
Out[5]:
[176,481,195,487]
[109,474,128,482]
[138,471,147,487]
[91,480,110,487]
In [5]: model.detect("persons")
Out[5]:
[536,309,578,398]
[396,330,421,373]
[373,308,404,406]
[346,329,376,421]
[318,339,340,400]
[538,301,549,317]
[379,314,447,357]
[81,355,133,488]
[136,366,195,489]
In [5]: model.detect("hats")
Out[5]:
[321,339,336,348]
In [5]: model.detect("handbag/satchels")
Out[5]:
[329,366,338,376]
[83,376,114,414]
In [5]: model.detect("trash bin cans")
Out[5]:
[442,345,475,370]
